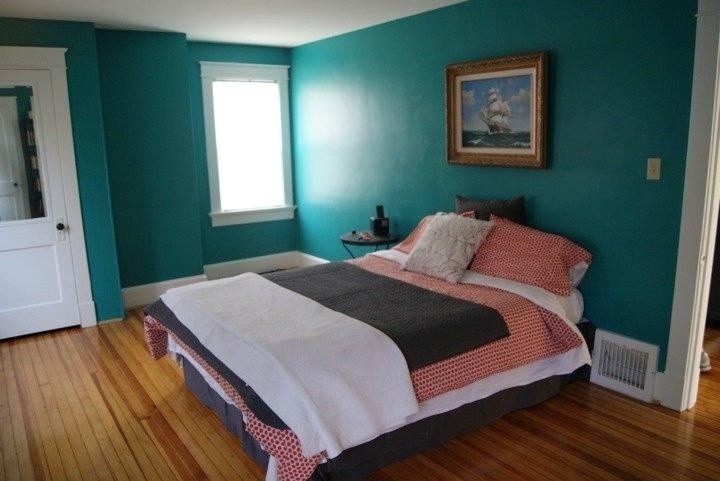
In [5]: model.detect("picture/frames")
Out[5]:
[447,51,550,172]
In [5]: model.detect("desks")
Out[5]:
[343,231,398,259]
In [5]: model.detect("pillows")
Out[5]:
[393,193,592,301]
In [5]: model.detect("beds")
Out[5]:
[142,211,593,481]
